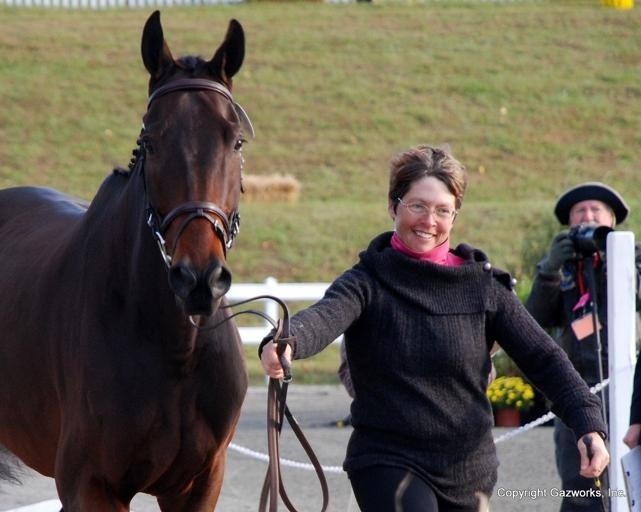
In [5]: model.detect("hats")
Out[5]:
[555,183,628,225]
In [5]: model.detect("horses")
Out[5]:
[0,10,248,512]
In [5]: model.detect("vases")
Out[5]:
[494,404,521,429]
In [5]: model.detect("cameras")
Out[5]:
[568,223,615,257]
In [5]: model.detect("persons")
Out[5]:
[257,145,610,512]
[624,350,641,450]
[524,183,641,512]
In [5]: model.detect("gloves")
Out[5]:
[542,231,578,274]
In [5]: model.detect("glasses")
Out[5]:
[397,198,455,221]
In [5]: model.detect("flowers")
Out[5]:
[483,370,538,413]
